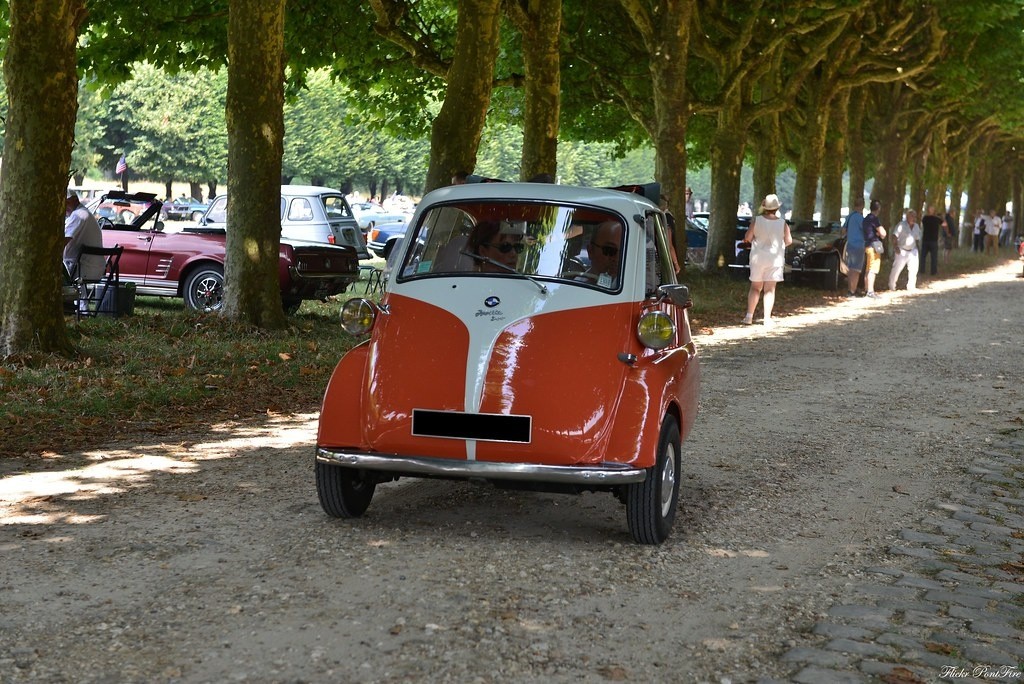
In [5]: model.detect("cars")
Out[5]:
[681,210,820,249]
[723,221,847,290]
[194,184,374,263]
[366,221,432,262]
[351,201,408,235]
[162,195,211,225]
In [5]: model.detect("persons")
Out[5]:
[451,173,466,185]
[348,191,394,208]
[942,210,956,265]
[63,190,107,284]
[998,211,1013,249]
[841,197,865,295]
[920,205,947,274]
[660,194,681,276]
[741,194,793,323]
[685,188,693,219]
[970,209,1002,257]
[888,209,921,291]
[972,209,986,253]
[462,220,523,275]
[862,200,886,298]
[571,221,623,290]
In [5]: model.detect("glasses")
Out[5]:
[483,242,524,254]
[591,240,620,257]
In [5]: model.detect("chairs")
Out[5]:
[433,234,481,273]
[60,244,124,322]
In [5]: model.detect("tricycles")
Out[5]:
[315,180,700,551]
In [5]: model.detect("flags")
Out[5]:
[116,157,126,174]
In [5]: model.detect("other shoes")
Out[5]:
[74,303,91,315]
[846,291,863,298]
[741,317,752,325]
[865,291,882,299]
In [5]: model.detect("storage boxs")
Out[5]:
[95,279,136,319]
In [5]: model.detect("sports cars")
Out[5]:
[79,186,363,320]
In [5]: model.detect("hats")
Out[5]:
[67,190,76,199]
[762,194,781,210]
[685,187,693,193]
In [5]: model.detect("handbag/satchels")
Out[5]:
[872,240,884,254]
